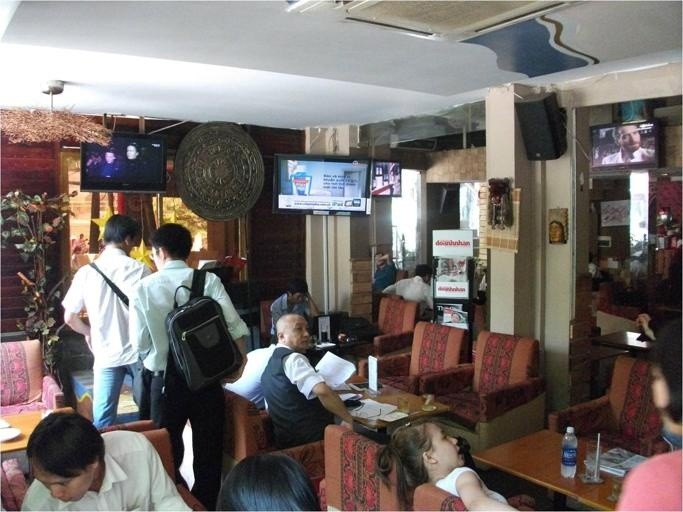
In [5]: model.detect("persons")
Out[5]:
[371,250,397,325]
[268,276,321,347]
[372,420,524,511]
[84,151,100,168]
[123,144,151,178]
[611,314,683,511]
[127,222,252,512]
[588,251,602,280]
[379,263,437,315]
[213,451,323,511]
[599,125,659,167]
[222,331,278,410]
[16,411,194,511]
[58,214,152,432]
[258,312,369,450]
[98,149,124,180]
[634,307,665,344]
[448,314,461,323]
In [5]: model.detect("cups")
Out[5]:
[612,475,623,500]
[586,441,600,479]
[422,394,434,409]
[396,396,410,413]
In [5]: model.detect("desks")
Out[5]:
[595,329,652,355]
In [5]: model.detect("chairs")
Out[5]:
[258,296,274,347]
[0,325,206,511]
[219,389,325,486]
[315,424,408,511]
[417,332,543,453]
[544,355,670,456]
[413,481,523,511]
[594,280,643,338]
[369,323,470,396]
[373,296,420,351]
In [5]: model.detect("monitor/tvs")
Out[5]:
[272,153,371,216]
[372,161,402,199]
[589,117,661,172]
[80,132,166,194]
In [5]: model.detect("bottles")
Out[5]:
[560,426,577,479]
[290,164,312,197]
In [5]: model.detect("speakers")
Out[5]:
[515,92,567,161]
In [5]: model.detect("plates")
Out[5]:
[0,428,21,442]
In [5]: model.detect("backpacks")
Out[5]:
[162,268,242,396]
